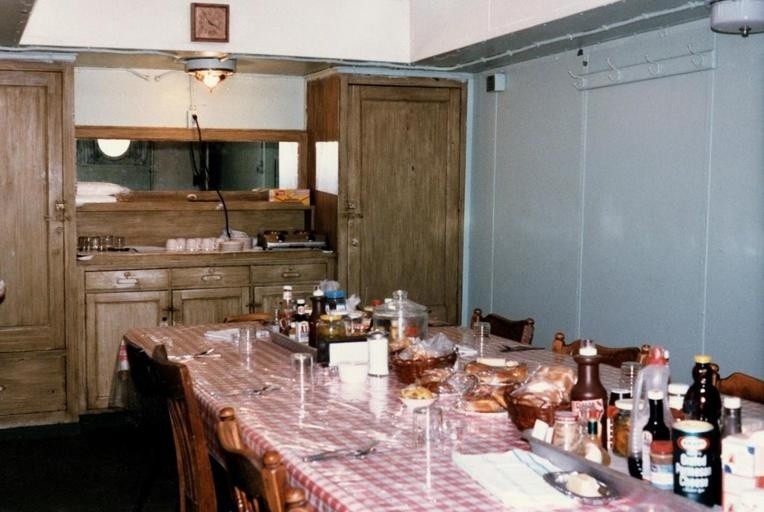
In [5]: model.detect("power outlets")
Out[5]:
[187,110,199,130]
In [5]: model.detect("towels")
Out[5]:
[451,448,580,508]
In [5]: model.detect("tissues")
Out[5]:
[328,341,369,399]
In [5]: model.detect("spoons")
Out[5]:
[494,343,546,353]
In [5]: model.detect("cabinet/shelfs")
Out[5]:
[1,60,77,429]
[305,71,468,329]
[251,255,334,310]
[76,261,250,416]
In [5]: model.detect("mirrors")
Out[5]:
[74,125,308,201]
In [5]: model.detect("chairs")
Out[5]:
[281,484,318,512]
[470,306,537,345]
[550,331,653,370]
[705,360,764,402]
[225,312,273,324]
[153,343,218,512]
[214,402,291,512]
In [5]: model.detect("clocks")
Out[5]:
[188,2,231,44]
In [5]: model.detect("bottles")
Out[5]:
[263,227,326,243]
[367,330,389,377]
[389,320,398,337]
[541,338,764,512]
[372,289,429,369]
[368,377,387,416]
[273,281,381,349]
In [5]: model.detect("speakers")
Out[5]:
[487,74,505,92]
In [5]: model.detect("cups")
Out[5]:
[164,235,226,253]
[290,352,314,389]
[471,317,490,356]
[78,233,124,251]
[238,324,256,354]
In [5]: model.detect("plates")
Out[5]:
[396,368,460,411]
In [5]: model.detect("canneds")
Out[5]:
[613,399,632,456]
[673,420,717,508]
[315,314,346,341]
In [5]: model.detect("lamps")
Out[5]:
[707,0,764,39]
[182,56,236,91]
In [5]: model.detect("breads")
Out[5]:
[402,349,575,413]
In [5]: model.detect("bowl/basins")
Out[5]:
[337,362,368,383]
[219,236,259,253]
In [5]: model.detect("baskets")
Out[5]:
[502,380,572,432]
[393,348,457,385]
